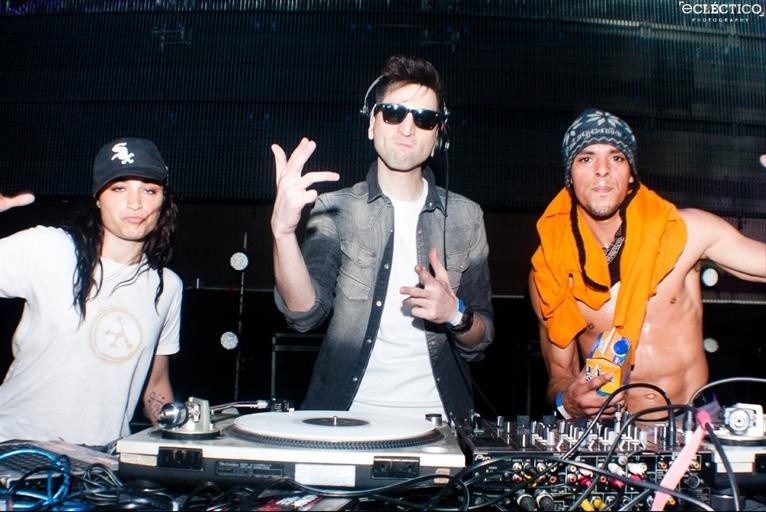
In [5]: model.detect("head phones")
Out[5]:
[360,72,452,158]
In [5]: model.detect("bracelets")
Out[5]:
[554,389,573,422]
[451,306,475,333]
[447,297,465,328]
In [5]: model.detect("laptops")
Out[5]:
[0,440,119,491]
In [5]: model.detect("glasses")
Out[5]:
[372,101,442,130]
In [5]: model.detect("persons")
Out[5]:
[524,101,766,446]
[270,56,496,426]
[0,135,186,447]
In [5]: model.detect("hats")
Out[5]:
[89,136,171,199]
[558,108,641,191]
